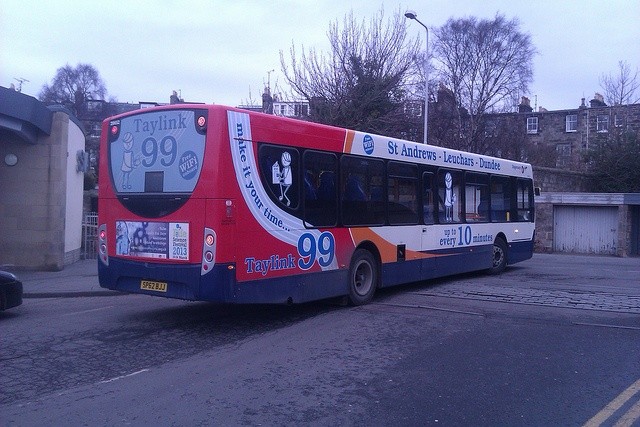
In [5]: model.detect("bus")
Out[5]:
[98,104,541,304]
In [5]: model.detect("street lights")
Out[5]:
[405,9,429,144]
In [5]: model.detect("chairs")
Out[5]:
[424,206,432,224]
[438,207,446,223]
[345,174,366,203]
[316,170,334,201]
[303,171,315,199]
